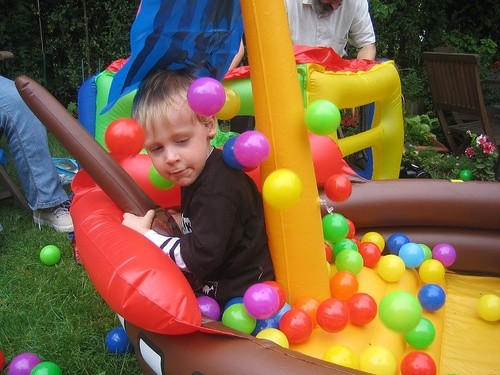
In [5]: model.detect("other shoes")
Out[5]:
[399,161,431,178]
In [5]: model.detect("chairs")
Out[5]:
[422,52,500,151]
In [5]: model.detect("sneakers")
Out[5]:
[32,202,74,233]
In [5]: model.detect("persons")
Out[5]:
[286,0,432,180]
[0,76,74,232]
[121,70,276,312]
